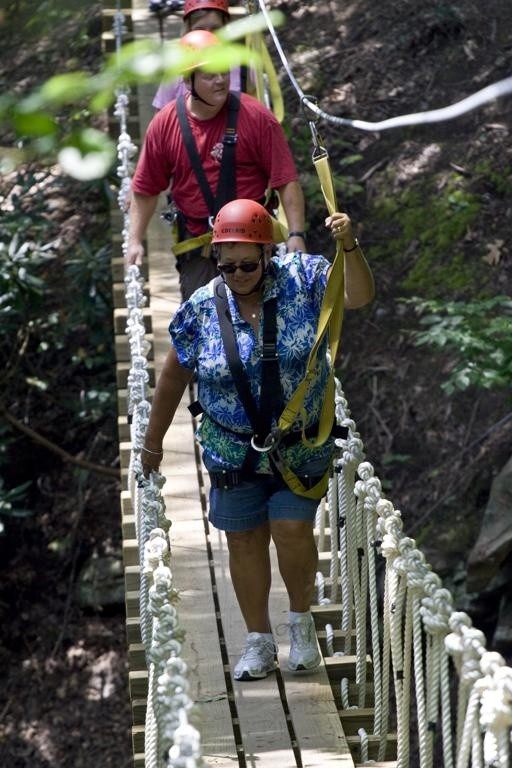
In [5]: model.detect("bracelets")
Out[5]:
[141,444,165,456]
[289,229,305,239]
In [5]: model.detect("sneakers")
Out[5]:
[286,615,321,671]
[149,0,165,12]
[234,632,280,680]
[166,0,184,10]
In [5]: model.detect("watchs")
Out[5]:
[340,238,360,253]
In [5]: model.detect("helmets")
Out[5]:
[183,0,230,22]
[175,31,224,74]
[210,199,273,244]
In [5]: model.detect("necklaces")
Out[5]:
[234,293,262,323]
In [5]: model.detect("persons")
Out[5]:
[137,198,378,682]
[122,30,309,308]
[152,0,261,115]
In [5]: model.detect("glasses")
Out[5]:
[216,262,258,274]
